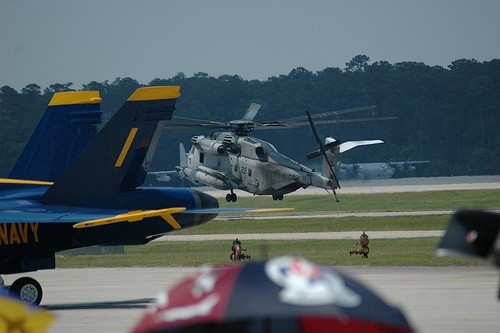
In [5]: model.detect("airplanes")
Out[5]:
[338,161,430,180]
[0,86,219,311]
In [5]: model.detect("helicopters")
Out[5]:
[165,103,397,202]
